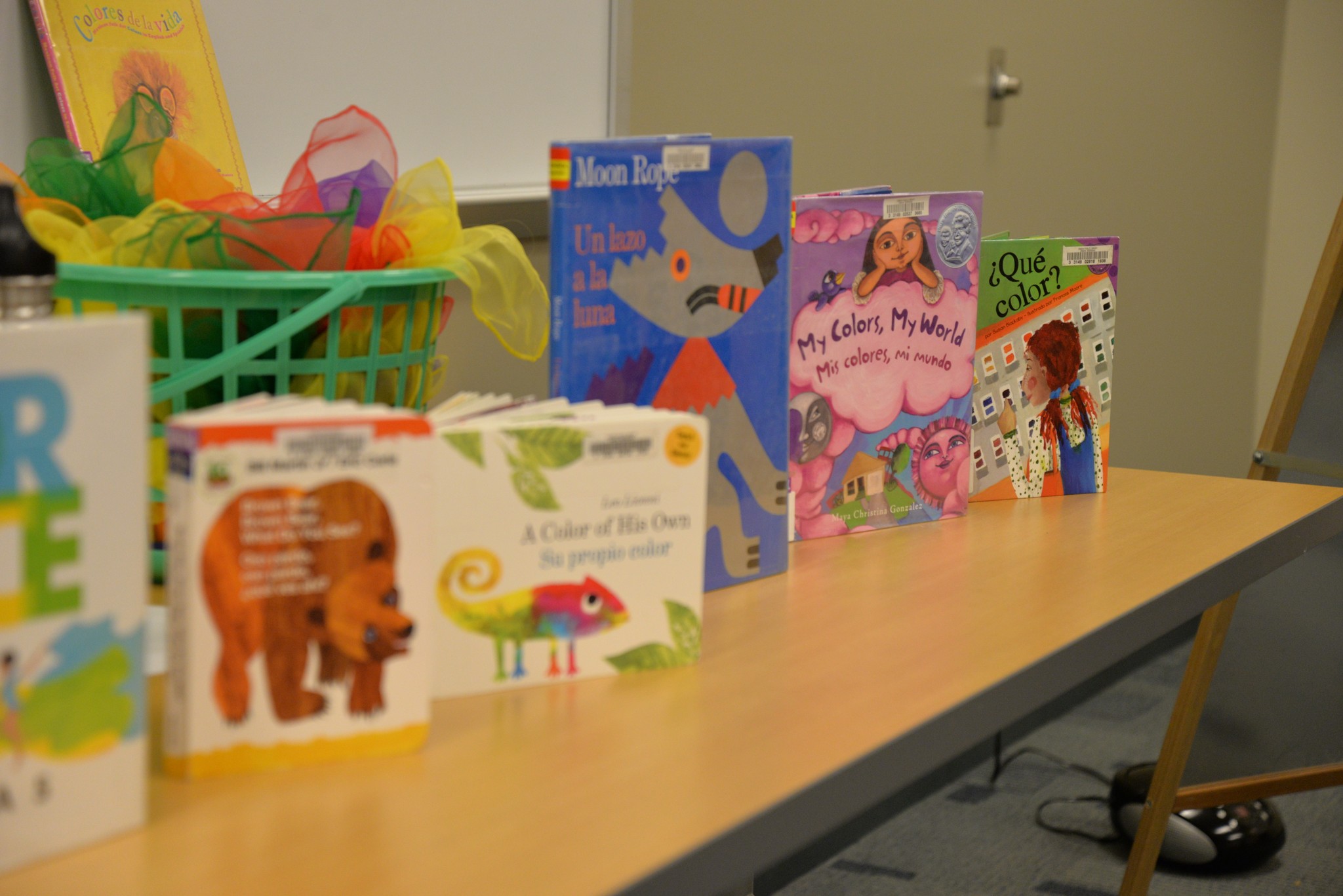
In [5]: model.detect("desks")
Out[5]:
[1,468,1342,896]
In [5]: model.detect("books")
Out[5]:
[546,132,794,595]
[165,389,437,781]
[787,186,983,541]
[971,232,1120,503]
[29,0,255,201]
[0,318,151,873]
[428,389,707,695]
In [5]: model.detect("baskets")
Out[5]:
[55,261,460,585]
